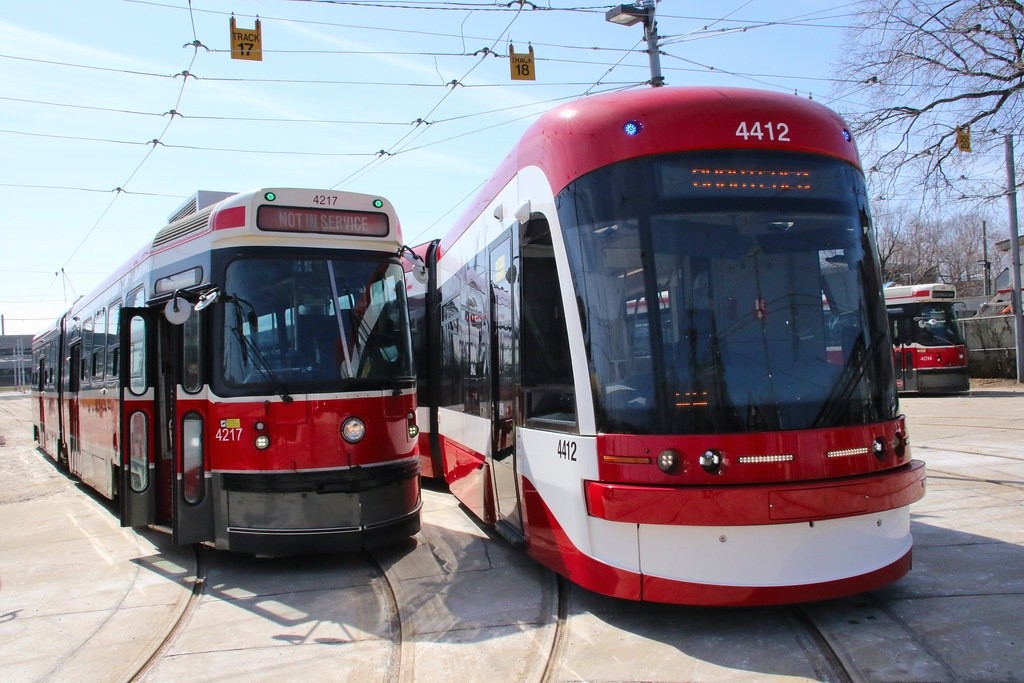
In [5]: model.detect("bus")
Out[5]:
[29,185,431,563]
[620,282,971,398]
[366,84,929,610]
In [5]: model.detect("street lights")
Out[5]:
[604,0,664,87]
